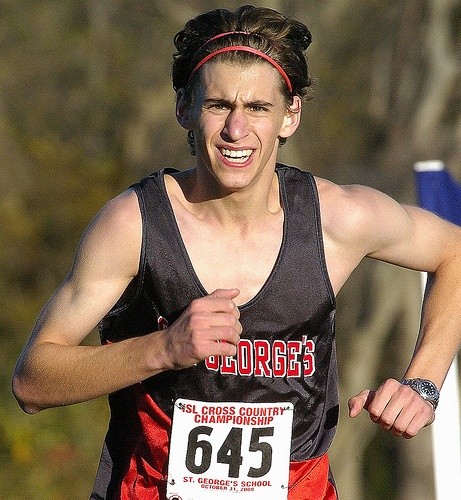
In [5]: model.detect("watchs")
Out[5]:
[399,378,440,411]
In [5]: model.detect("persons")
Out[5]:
[11,5,461,500]
